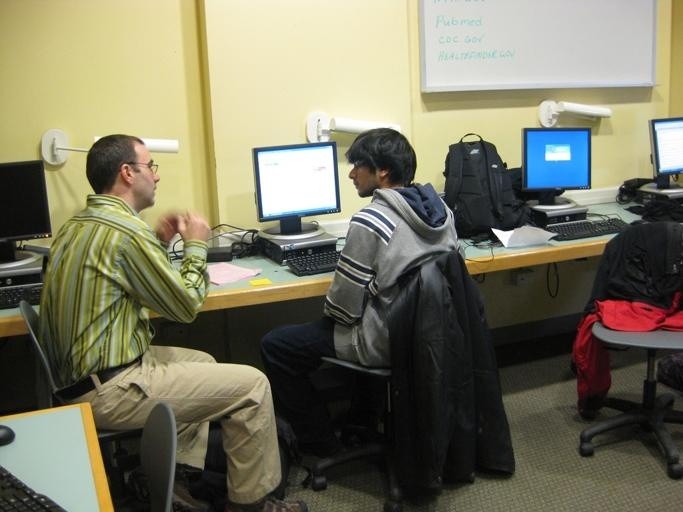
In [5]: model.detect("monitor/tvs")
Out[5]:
[250,141,343,240]
[520,127,592,209]
[637,117,682,194]
[0,159,52,268]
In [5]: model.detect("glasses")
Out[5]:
[127,160,158,174]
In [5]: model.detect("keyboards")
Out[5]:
[0,464,68,512]
[542,216,632,241]
[286,249,342,277]
[1,283,45,310]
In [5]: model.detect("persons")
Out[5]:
[261,128,460,468]
[39,135,308,512]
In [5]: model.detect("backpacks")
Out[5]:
[442,133,525,241]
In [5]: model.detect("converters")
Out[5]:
[204,247,233,263]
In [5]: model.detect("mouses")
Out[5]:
[630,219,650,224]
[1,423,15,447]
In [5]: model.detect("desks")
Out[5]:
[1,401,113,512]
[0,200,643,339]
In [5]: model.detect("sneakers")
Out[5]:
[127,467,210,512]
[313,433,338,457]
[224,496,307,511]
[338,426,360,446]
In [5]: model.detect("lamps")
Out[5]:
[42,129,180,165]
[538,98,612,127]
[307,110,402,143]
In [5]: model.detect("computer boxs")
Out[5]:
[525,196,589,229]
[0,253,48,310]
[252,227,339,266]
[632,189,683,206]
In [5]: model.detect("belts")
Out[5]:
[50,355,138,402]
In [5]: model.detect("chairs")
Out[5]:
[19,300,127,439]
[309,251,455,511]
[576,222,683,481]
[138,402,177,511]
